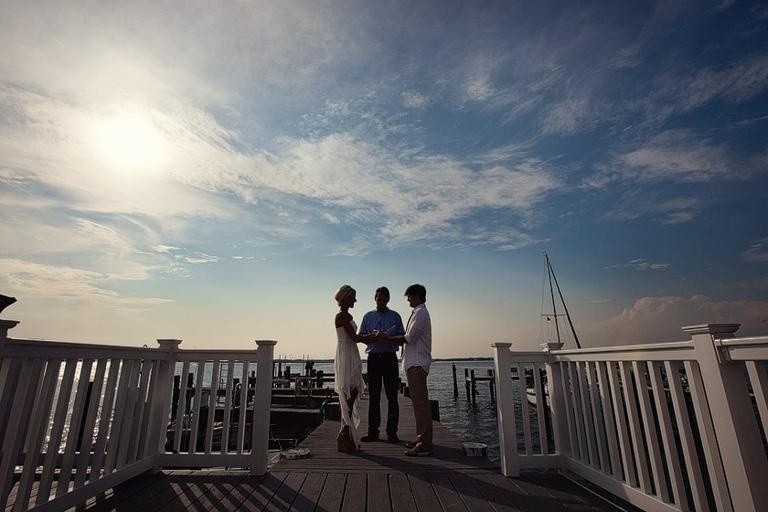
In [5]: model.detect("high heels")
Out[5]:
[335,431,361,451]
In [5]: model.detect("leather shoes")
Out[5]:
[360,435,379,442]
[388,434,399,443]
[404,435,433,456]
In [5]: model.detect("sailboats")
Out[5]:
[524,250,623,409]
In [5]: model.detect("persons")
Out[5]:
[333,285,377,452]
[377,283,434,456]
[359,287,405,442]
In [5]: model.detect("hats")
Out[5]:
[335,284,355,306]
